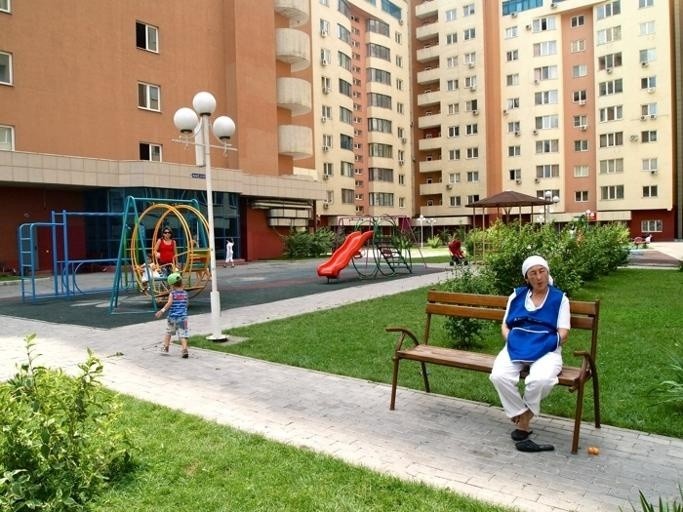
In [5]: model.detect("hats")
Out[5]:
[168,274,182,285]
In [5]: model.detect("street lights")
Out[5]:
[426,217,436,240]
[535,190,559,222]
[168,90,241,345]
[415,215,426,248]
[580,208,594,227]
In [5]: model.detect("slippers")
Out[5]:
[516,440,554,452]
[511,429,533,442]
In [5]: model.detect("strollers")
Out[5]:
[447,238,468,267]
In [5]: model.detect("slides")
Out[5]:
[318,231,373,276]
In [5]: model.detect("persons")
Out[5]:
[141,253,155,297]
[154,227,178,300]
[489,255,572,441]
[224,238,235,268]
[156,273,189,358]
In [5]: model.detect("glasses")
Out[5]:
[164,232,171,234]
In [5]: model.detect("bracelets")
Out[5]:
[161,308,166,313]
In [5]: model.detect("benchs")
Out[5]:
[382,287,602,456]
